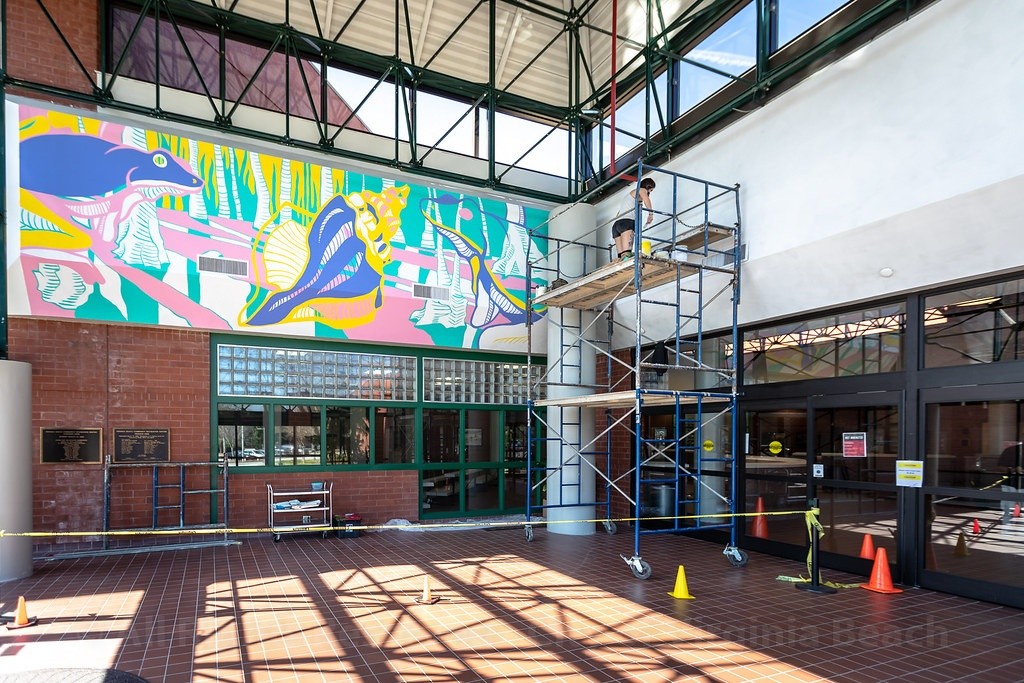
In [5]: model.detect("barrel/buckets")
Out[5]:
[640,241,688,262]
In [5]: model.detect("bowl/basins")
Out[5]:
[312,482,323,489]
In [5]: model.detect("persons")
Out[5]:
[612,178,655,259]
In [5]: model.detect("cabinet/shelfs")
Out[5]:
[267,481,333,542]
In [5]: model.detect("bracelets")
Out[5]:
[649,214,653,215]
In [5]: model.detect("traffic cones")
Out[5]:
[859,533,876,560]
[667,564,696,599]
[6,596,37,629]
[950,532,971,557]
[415,575,440,604]
[971,518,982,534]
[750,496,769,539]
[859,547,904,593]
[1011,504,1021,518]
